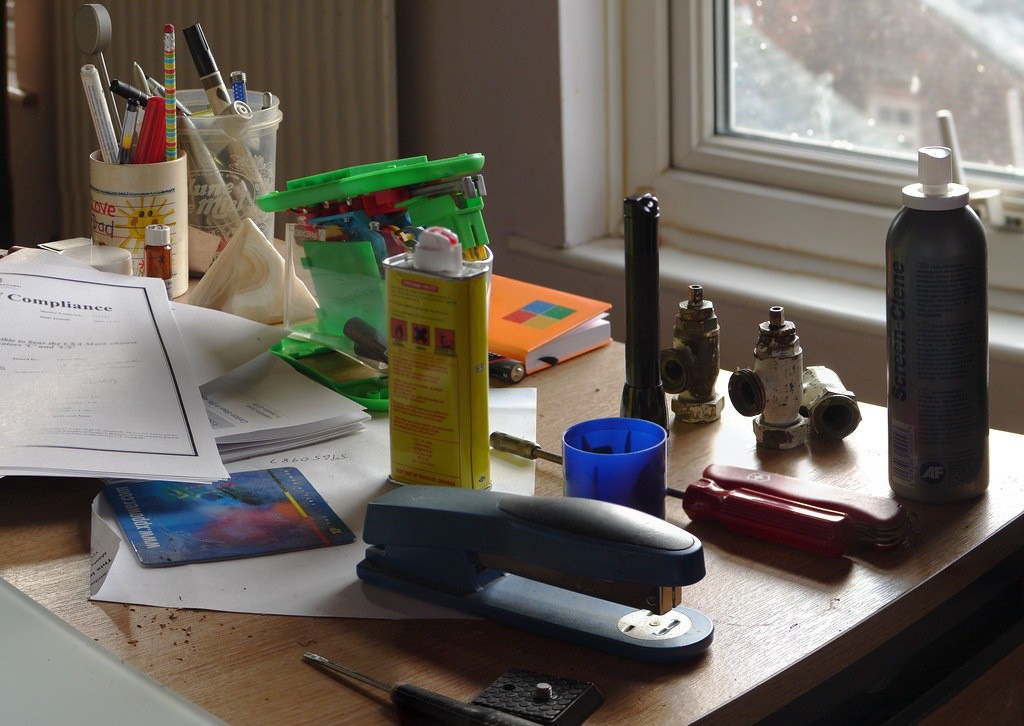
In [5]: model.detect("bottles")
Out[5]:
[144,224,174,301]
[882,146,993,506]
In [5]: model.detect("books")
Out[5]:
[488,274,612,375]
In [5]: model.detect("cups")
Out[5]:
[165,87,283,274]
[558,418,668,517]
[58,244,132,278]
[88,149,190,297]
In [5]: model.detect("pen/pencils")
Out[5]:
[170,22,277,275]
[81,24,191,295]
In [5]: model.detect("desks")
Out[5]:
[1,237,1023,726]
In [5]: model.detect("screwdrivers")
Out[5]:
[305,654,540,726]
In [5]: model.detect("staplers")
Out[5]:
[682,463,914,564]
[357,483,715,669]
